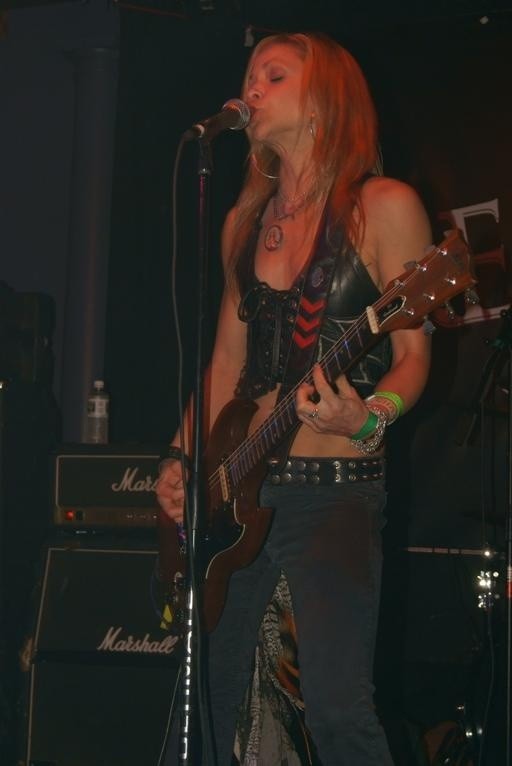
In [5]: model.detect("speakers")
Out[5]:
[27,544,187,766]
[377,315,512,766]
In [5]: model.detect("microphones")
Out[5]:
[182,98,252,142]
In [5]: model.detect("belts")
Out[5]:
[267,454,384,485]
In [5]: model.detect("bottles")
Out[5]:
[88,380,109,446]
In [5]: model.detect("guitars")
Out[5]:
[159,229,472,632]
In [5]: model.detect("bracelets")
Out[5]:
[349,410,376,440]
[368,395,397,426]
[372,390,406,420]
[158,444,196,470]
[354,404,385,451]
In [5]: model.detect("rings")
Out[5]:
[308,406,319,420]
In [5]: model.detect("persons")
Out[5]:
[155,32,436,766]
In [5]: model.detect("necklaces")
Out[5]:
[266,186,321,249]
